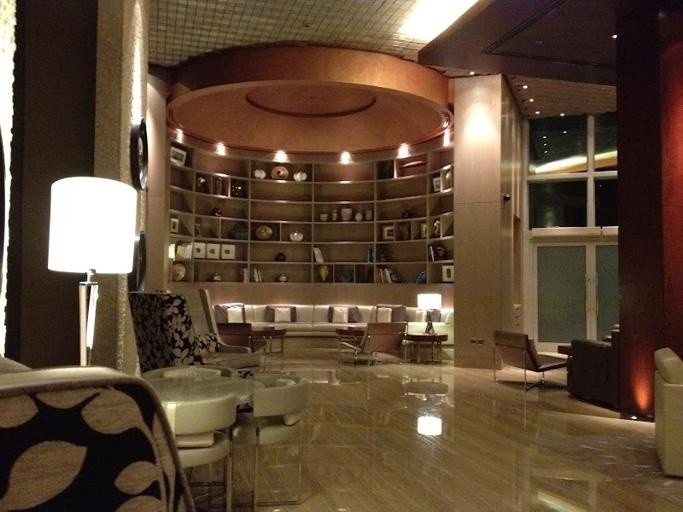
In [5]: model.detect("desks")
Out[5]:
[558,346,573,392]
[336,328,365,353]
[404,332,447,363]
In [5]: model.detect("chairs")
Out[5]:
[340,323,407,366]
[492,331,570,392]
[656,347,683,479]
[568,323,620,410]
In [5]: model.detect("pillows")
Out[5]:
[214,303,450,323]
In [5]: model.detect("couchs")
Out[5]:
[0,355,196,512]
[251,311,455,346]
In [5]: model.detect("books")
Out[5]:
[251,266,265,282]
[378,266,399,284]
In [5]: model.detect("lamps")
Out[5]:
[417,411,442,436]
[48,176,137,368]
[417,293,441,334]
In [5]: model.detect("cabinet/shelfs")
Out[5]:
[166,136,454,285]
